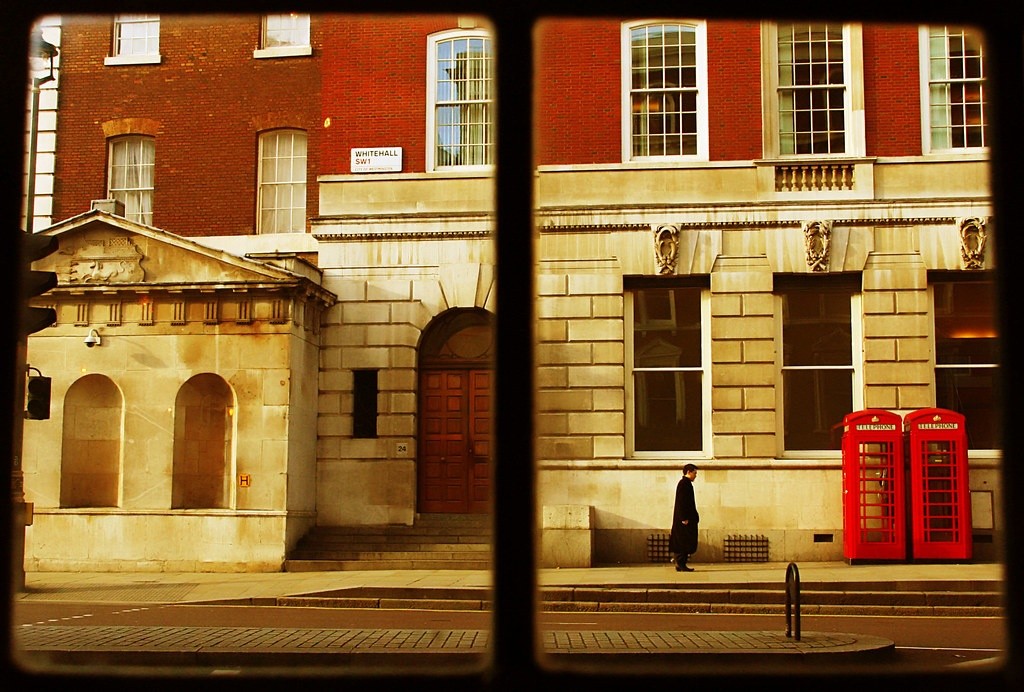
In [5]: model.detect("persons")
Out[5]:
[669,464,699,572]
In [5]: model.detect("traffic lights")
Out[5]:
[26,377,51,420]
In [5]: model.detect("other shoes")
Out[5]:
[676,565,694,571]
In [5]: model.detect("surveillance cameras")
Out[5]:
[84,337,97,347]
